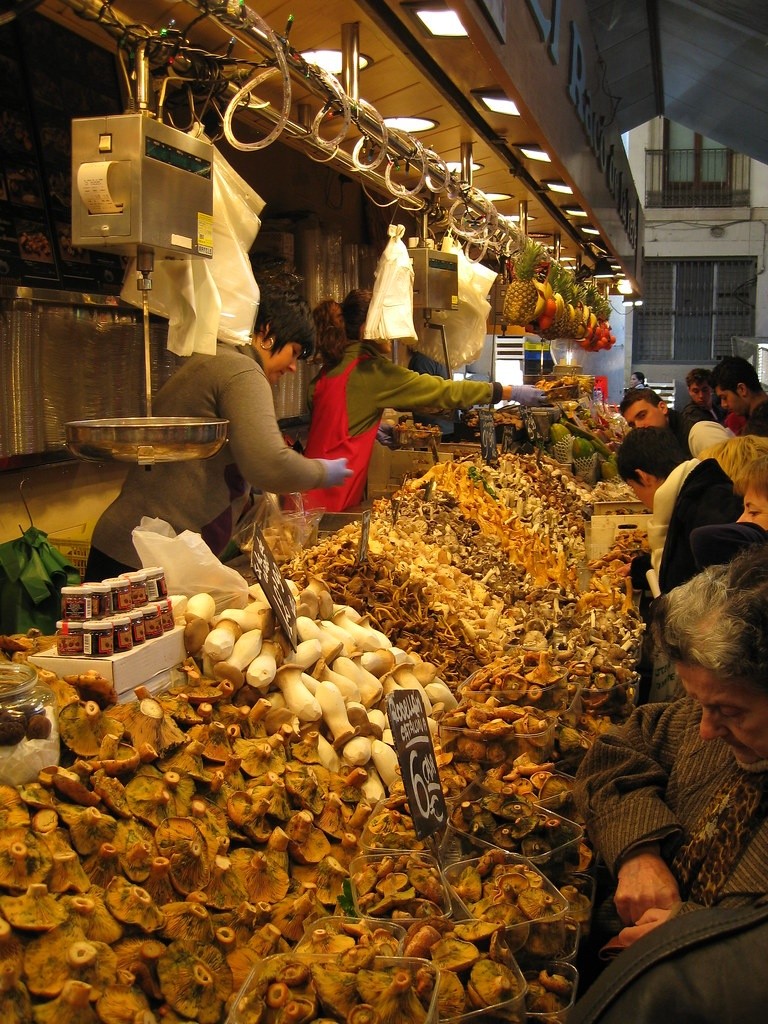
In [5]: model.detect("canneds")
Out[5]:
[83,621,114,657]
[61,586,93,620]
[115,612,145,645]
[102,579,132,611]
[82,583,111,618]
[137,567,167,600]
[149,600,175,630]
[102,617,133,651]
[134,606,163,637]
[119,574,149,606]
[55,621,84,655]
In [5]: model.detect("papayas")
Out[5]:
[551,423,617,482]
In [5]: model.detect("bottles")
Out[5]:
[0,661,60,788]
[593,387,602,401]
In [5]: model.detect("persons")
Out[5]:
[574,388,768,1024]
[629,372,649,389]
[408,351,455,443]
[284,288,549,515]
[680,367,725,439]
[87,271,353,582]
[708,355,768,437]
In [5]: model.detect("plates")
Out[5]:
[63,417,230,463]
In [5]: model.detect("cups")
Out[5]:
[584,520,617,559]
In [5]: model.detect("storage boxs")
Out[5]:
[584,501,654,562]
[26,624,187,707]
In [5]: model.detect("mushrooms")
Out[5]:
[0,450,649,1023]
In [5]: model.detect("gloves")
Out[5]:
[508,383,550,407]
[317,457,353,489]
[376,422,398,446]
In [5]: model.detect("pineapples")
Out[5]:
[504,237,610,340]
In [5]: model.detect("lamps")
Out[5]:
[400,0,643,308]
